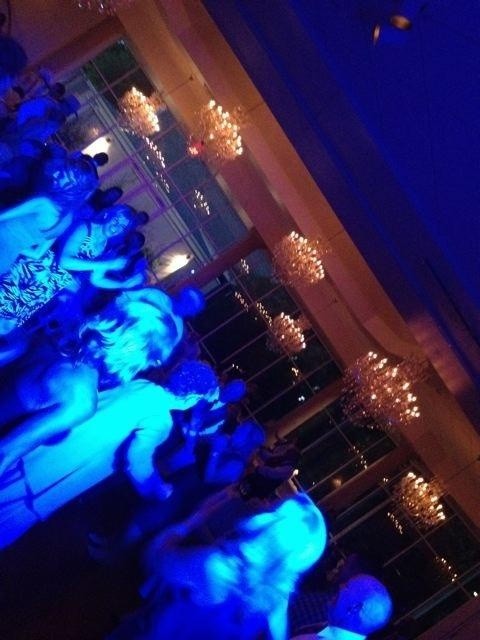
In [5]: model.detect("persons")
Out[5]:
[12,57,397,634]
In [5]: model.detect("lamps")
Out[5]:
[118,76,480,584]
